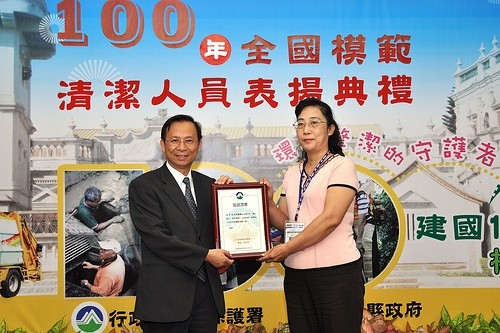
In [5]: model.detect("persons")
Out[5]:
[77,187,125,232]
[128,114,235,333]
[81,238,125,297]
[255,98,364,333]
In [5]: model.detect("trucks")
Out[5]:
[0,209,45,299]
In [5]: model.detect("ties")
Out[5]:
[182,176,207,283]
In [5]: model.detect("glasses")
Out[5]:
[294,119,325,128]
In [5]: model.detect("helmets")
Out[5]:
[84,186,101,201]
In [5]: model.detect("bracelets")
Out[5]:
[85,282,88,285]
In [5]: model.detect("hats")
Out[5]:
[96,238,122,254]
